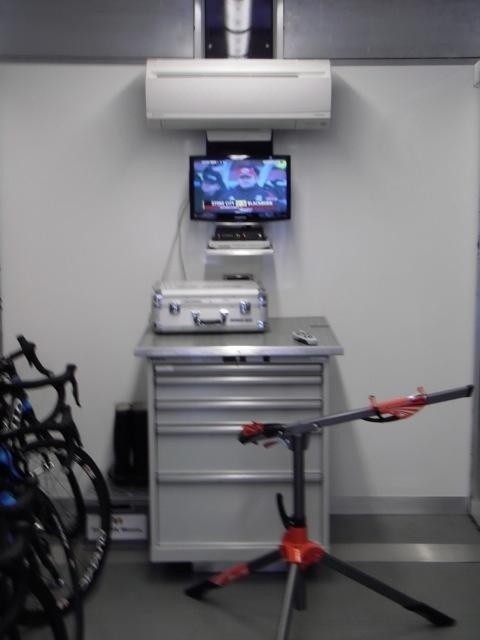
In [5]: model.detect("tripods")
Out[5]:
[182,383,474,640]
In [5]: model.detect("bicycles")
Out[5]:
[0,335,111,640]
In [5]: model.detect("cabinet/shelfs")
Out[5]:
[134,316,346,573]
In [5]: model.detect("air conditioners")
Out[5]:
[144,55,331,134]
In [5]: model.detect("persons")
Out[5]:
[193,160,228,214]
[222,165,278,211]
[263,160,287,211]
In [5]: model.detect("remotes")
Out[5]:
[292,329,317,345]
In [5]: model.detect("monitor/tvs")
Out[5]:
[189,154,292,228]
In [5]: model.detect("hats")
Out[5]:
[203,170,222,184]
[238,167,255,177]
